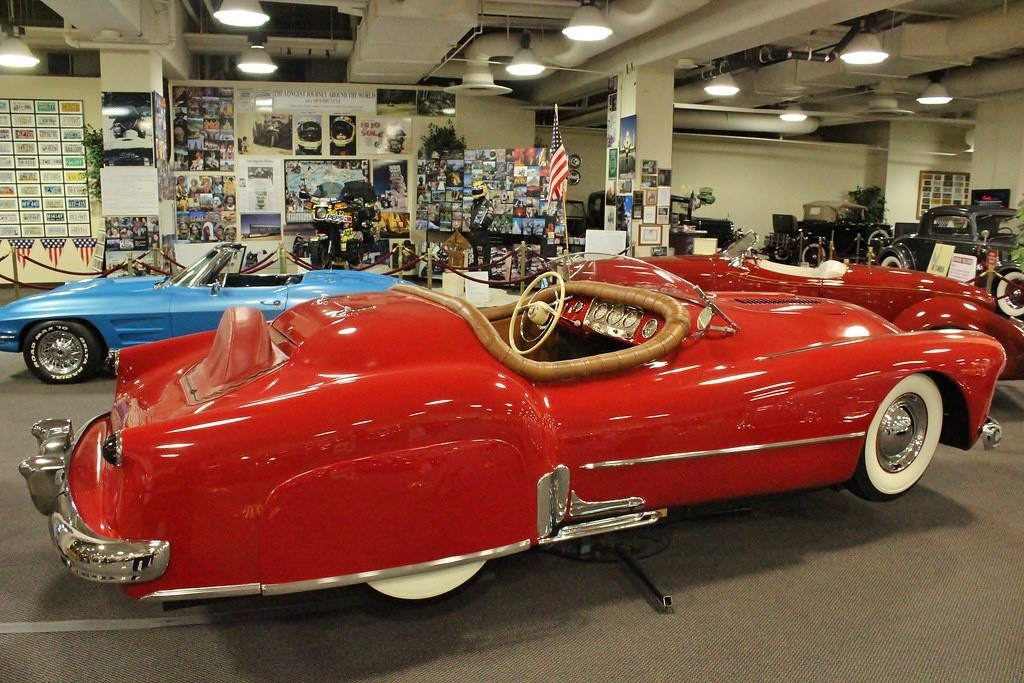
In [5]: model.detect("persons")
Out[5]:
[101,80,564,291]
[606,143,672,257]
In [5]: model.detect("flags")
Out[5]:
[546,104,570,212]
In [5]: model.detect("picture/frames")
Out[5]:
[0,98,92,239]
[631,158,670,247]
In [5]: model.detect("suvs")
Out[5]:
[587,190,736,255]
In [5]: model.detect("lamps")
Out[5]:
[505,34,546,76]
[1,25,40,69]
[779,104,808,121]
[561,0,614,41]
[837,15,890,65]
[212,0,270,27]
[235,34,279,74]
[915,70,953,105]
[703,71,741,95]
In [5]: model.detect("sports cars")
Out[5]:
[18,252,1008,619]
[1,242,413,384]
[563,230,1024,382]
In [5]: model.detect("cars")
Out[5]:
[761,200,894,266]
[878,204,1024,319]
